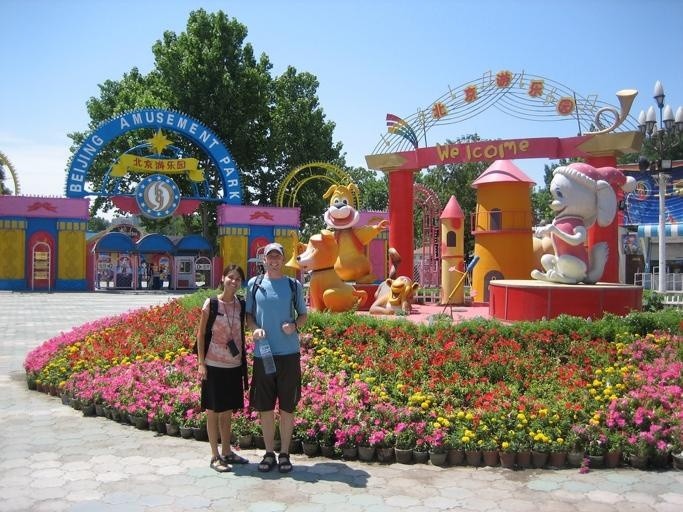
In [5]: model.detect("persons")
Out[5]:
[196,264,249,472]
[245,242,307,473]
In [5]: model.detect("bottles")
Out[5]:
[258,336,277,376]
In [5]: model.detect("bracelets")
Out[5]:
[294,322,297,328]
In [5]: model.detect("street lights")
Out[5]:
[637,81,683,295]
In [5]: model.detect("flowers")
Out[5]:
[274,397,281,418]
[24,299,208,424]
[292,308,683,472]
[242,331,263,429]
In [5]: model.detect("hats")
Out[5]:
[263,242,285,256]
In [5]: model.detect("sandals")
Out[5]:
[278,453,292,474]
[221,452,249,464]
[257,452,277,472]
[210,455,231,473]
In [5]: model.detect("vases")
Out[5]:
[149,423,208,442]
[273,440,281,452]
[240,434,267,450]
